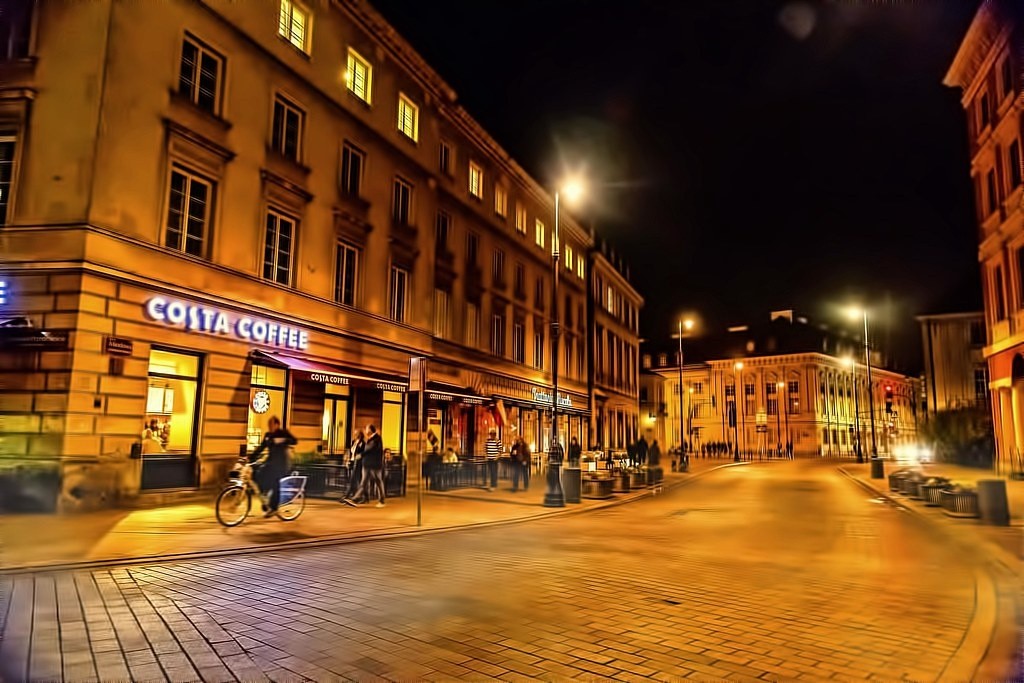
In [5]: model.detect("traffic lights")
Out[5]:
[886,403,892,413]
[884,386,892,398]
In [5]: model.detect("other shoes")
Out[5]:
[344,498,357,506]
[376,502,386,508]
[265,509,278,517]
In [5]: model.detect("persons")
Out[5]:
[142,419,170,453]
[340,424,385,507]
[510,439,531,491]
[240,417,297,518]
[627,434,660,467]
[701,440,732,458]
[778,440,794,460]
[548,439,564,465]
[568,437,581,467]
[426,446,457,488]
[483,431,502,491]
[385,449,391,460]
[668,446,679,470]
[427,431,440,452]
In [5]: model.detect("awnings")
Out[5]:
[254,349,493,405]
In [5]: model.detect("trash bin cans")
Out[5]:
[870,459,884,479]
[563,468,581,503]
[977,478,1009,528]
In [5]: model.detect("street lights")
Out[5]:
[843,359,863,463]
[677,318,693,467]
[733,362,744,460]
[850,308,877,459]
[776,381,784,456]
[552,176,583,442]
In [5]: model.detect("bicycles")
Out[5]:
[216,460,304,527]
